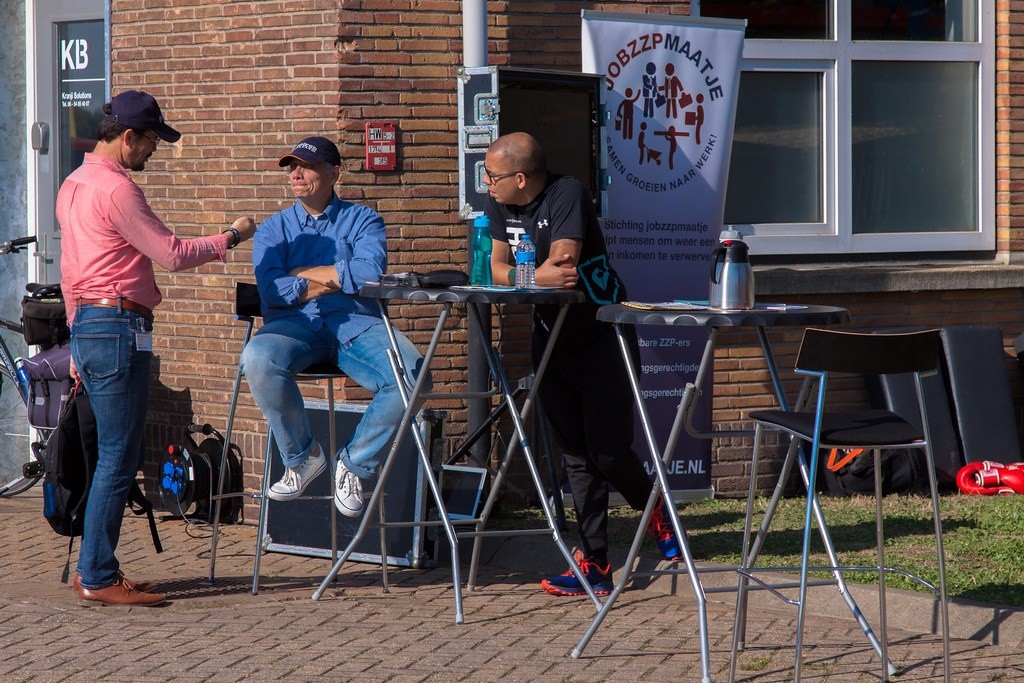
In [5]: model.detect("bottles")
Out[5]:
[515,235,537,290]
[469,216,496,284]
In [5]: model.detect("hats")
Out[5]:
[279,137,341,168]
[105,90,181,143]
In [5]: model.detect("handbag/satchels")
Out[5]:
[23,342,77,429]
[22,286,70,346]
[803,439,928,496]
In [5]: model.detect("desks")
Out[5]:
[310,273,605,624]
[571,297,896,683]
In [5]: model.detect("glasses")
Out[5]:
[484,161,531,185]
[123,127,161,146]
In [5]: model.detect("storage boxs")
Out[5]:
[258,401,449,571]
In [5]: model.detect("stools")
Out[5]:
[204,278,393,581]
[728,326,950,683]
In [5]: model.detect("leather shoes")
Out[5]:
[78,574,165,607]
[73,567,158,593]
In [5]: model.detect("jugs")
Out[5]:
[708,238,757,308]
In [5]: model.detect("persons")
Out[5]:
[241,136,434,517]
[56,91,256,607]
[477,131,683,596]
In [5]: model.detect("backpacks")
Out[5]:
[42,380,100,537]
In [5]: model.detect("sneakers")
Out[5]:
[332,448,365,517]
[269,443,328,501]
[542,549,613,596]
[646,492,688,561]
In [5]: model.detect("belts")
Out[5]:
[75,297,153,317]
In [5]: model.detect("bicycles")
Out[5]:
[0,234,70,500]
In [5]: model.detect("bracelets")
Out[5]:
[222,229,239,250]
